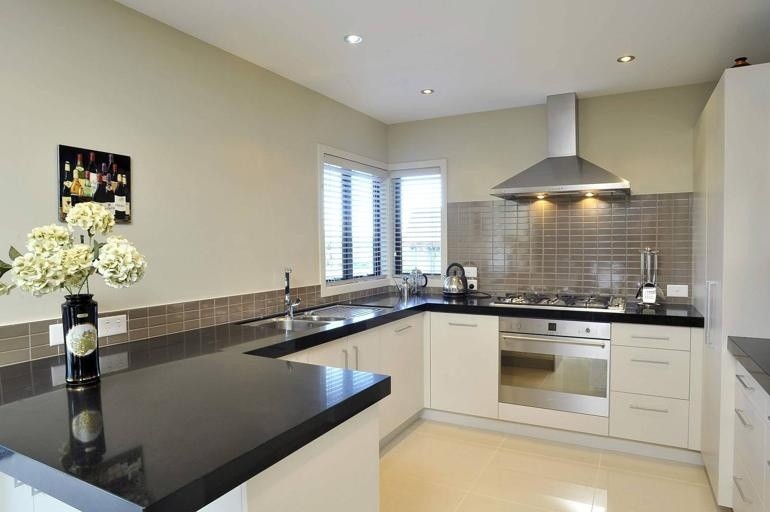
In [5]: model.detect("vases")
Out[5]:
[66,379,107,470]
[61,295,102,382]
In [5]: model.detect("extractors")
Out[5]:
[489,91,633,201]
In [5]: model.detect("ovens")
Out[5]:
[498,331,612,421]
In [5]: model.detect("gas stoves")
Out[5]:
[490,293,626,313]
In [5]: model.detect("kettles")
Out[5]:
[410,266,428,294]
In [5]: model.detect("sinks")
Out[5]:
[295,315,347,322]
[241,317,331,333]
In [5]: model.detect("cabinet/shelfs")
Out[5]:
[608,320,693,453]
[730,360,770,511]
[691,61,770,511]
[309,326,386,446]
[423,310,500,415]
[386,310,424,435]
[0,471,83,511]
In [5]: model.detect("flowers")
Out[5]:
[0,200,149,295]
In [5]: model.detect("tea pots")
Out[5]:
[440,262,468,299]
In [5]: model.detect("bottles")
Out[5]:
[400,276,411,297]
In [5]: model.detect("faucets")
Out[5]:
[284,267,302,319]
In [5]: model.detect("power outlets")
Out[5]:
[467,280,477,291]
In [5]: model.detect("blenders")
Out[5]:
[635,245,665,307]
